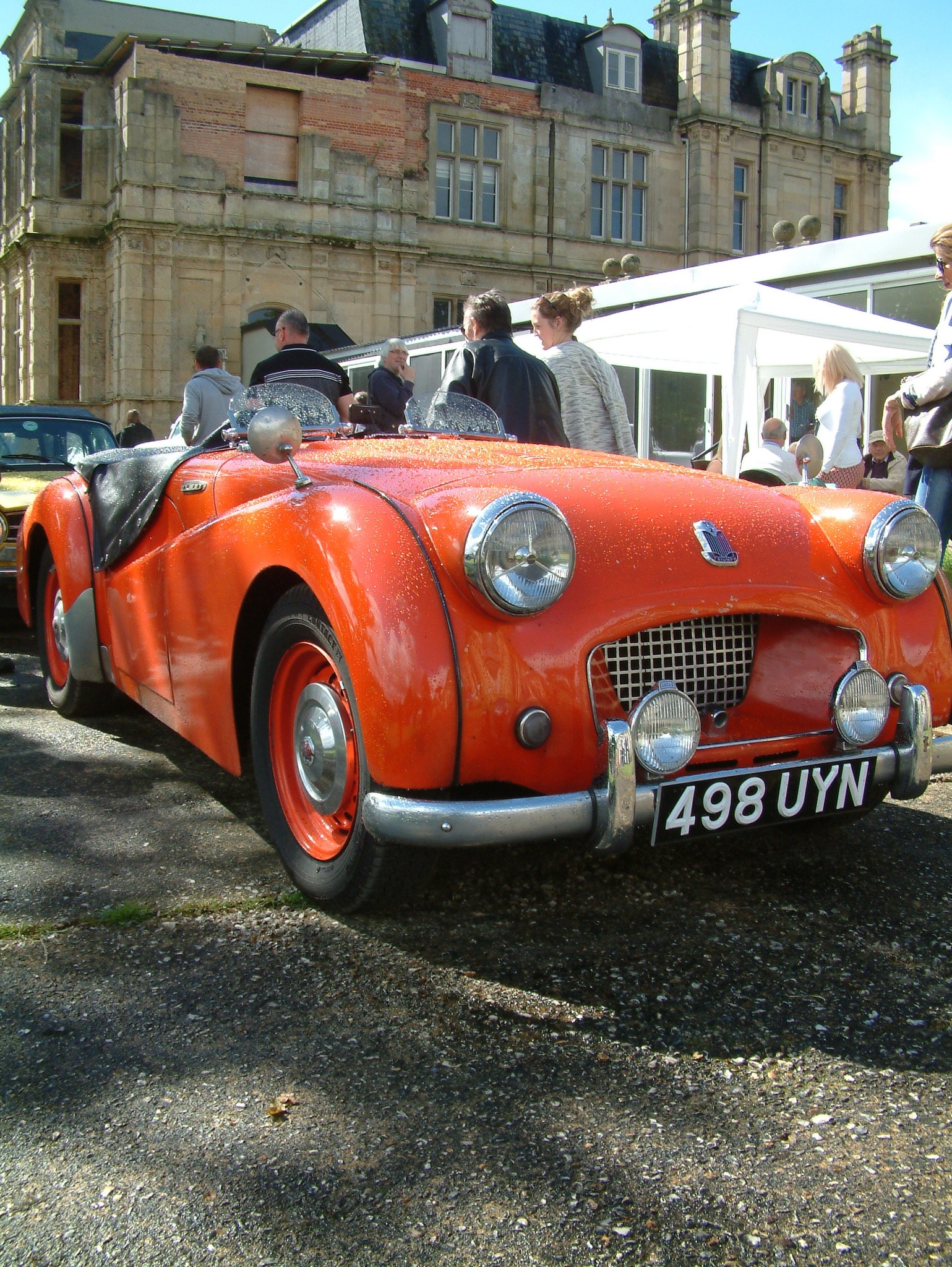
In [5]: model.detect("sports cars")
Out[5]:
[19,383,952,918]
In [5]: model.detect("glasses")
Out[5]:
[935,257,951,275]
[459,318,473,335]
[388,349,408,358]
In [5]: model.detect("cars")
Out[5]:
[0,402,123,578]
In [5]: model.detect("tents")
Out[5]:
[513,266,935,478]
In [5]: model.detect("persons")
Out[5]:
[368,338,425,430]
[114,409,154,448]
[857,429,908,496]
[530,286,638,456]
[428,288,569,446]
[249,310,356,422]
[813,343,866,488]
[707,430,746,475]
[354,391,368,405]
[791,380,813,441]
[180,346,247,446]
[883,224,952,569]
[788,442,800,455]
[741,418,801,483]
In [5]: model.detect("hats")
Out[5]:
[868,429,886,444]
